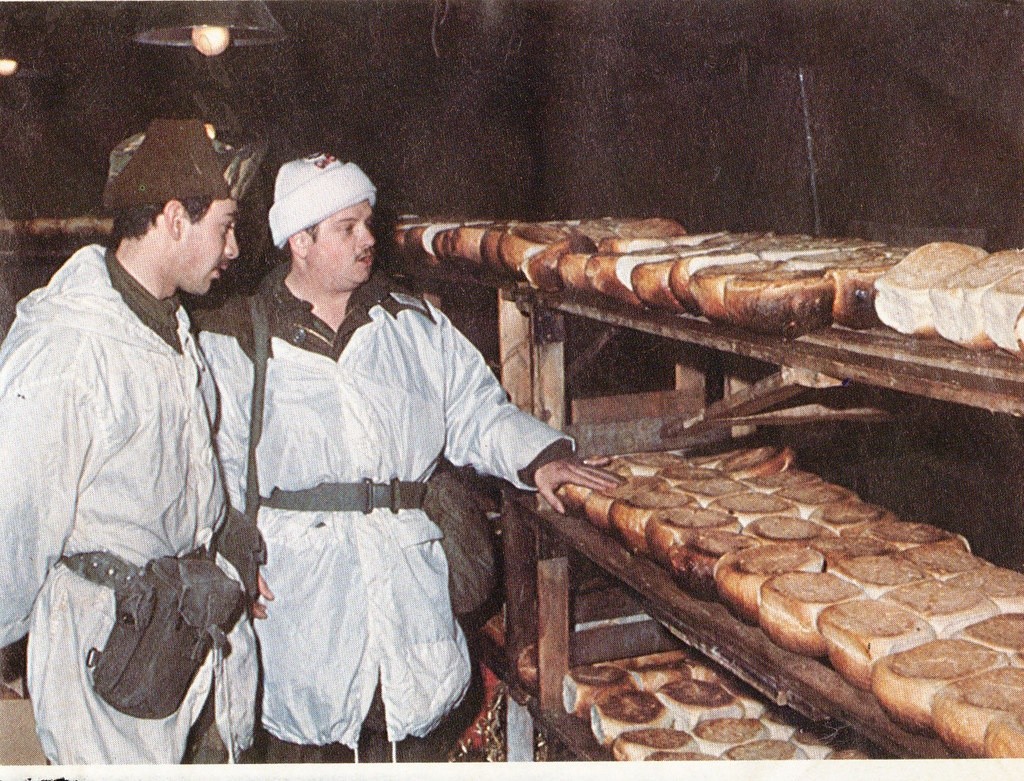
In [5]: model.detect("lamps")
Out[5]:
[130,0,289,56]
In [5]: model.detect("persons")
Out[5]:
[0,122,262,765]
[227,150,626,762]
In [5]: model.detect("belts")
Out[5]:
[257,482,427,516]
[59,546,210,588]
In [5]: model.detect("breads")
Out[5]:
[377,215,1024,361]
[0,214,116,238]
[518,441,1024,759]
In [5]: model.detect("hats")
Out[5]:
[103,120,266,217]
[268,151,379,249]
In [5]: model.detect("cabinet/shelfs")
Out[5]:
[382,259,1024,763]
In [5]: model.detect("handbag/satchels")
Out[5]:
[422,472,499,614]
[85,557,248,721]
